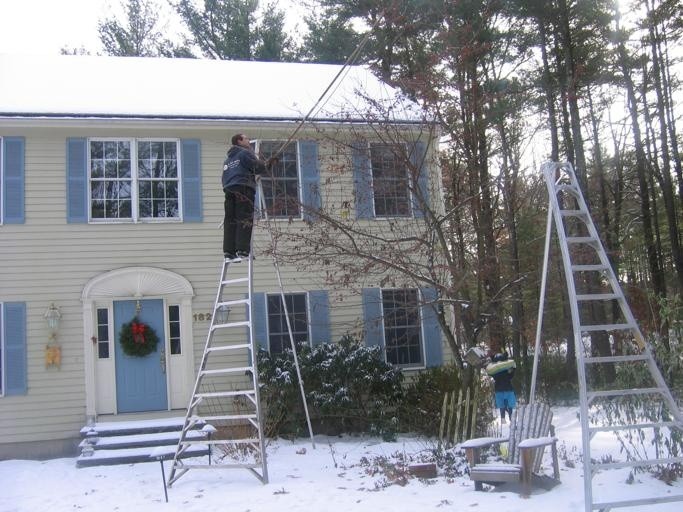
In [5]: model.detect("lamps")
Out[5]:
[43,302,62,337]
[215,302,231,325]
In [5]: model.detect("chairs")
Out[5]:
[460,401,563,499]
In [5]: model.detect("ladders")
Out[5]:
[167,140,316,488]
[529,161,683,512]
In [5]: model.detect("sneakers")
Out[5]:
[223,251,255,263]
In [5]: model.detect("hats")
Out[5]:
[494,354,505,361]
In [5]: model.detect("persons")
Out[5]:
[486,353,518,425]
[222,134,280,264]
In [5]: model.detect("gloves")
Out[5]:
[268,158,279,166]
